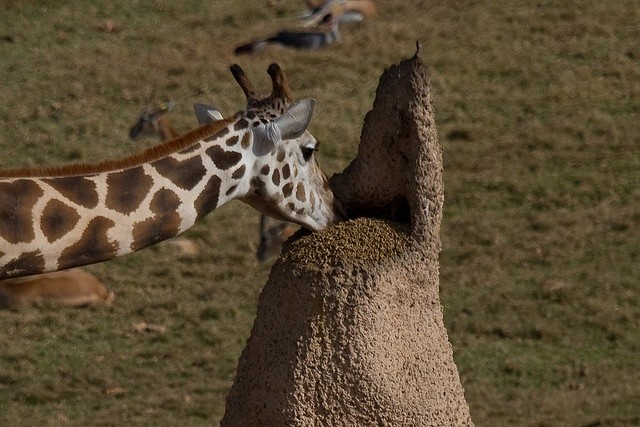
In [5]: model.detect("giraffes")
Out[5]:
[1,62,352,281]
